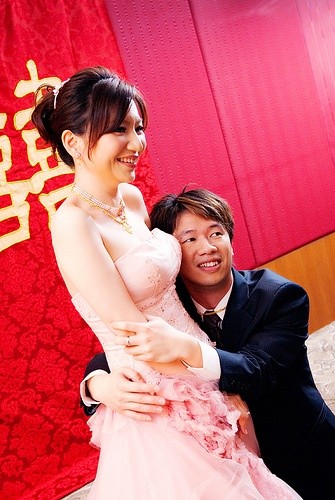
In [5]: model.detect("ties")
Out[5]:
[199,309,224,343]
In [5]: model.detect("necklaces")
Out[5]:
[197,306,226,316]
[71,182,134,234]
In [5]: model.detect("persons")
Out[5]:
[30,66,304,500]
[79,183,335,500]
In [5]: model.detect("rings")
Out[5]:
[127,335,131,346]
